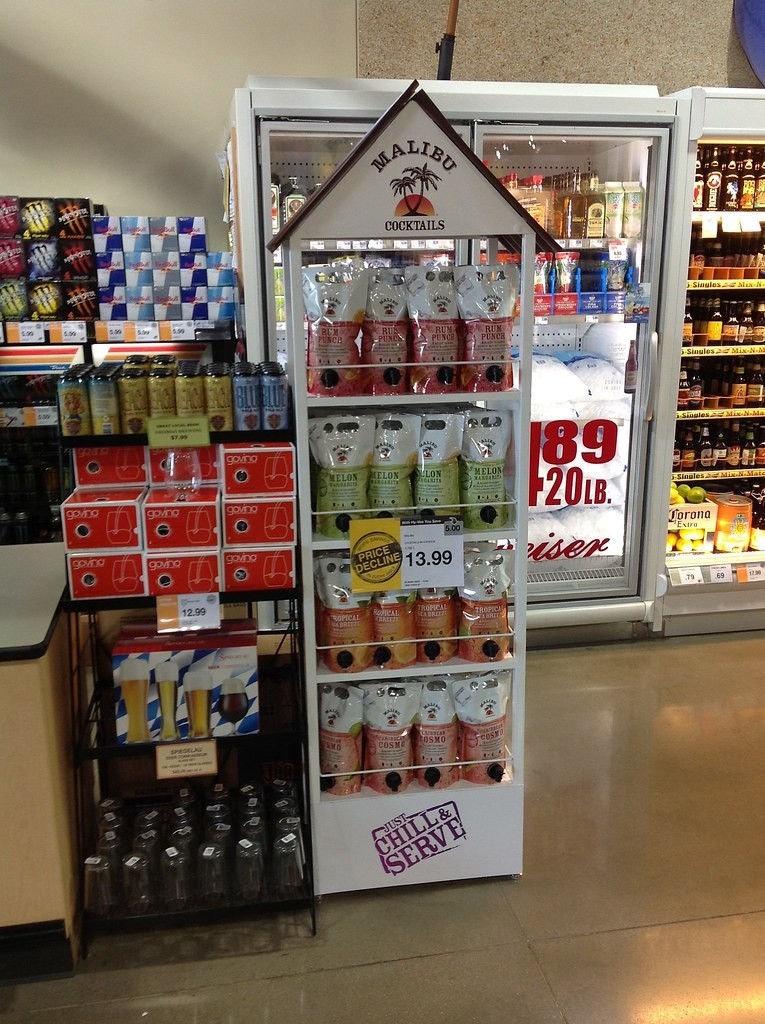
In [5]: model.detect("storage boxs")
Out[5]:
[60,443,300,600]
[0,195,240,320]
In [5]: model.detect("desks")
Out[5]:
[1,543,91,983]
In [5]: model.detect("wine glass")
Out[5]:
[218,679,249,736]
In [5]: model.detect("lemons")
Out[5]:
[662,482,708,552]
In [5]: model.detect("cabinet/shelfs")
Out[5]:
[56,392,318,958]
[265,78,564,902]
[651,85,765,637]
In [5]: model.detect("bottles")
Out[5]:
[693,146,764,210]
[501,168,604,240]
[688,220,765,281]
[272,174,324,233]
[677,355,764,410]
[673,420,765,471]
[625,339,639,393]
[734,479,765,551]
[682,297,765,347]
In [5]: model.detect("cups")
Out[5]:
[166,450,201,501]
[83,778,307,916]
[119,658,213,744]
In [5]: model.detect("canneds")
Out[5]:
[702,483,753,553]
[56,356,288,437]
